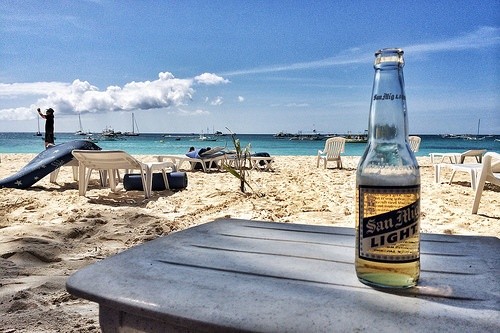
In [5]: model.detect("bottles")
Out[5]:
[126,200,134,204]
[355,46,422,290]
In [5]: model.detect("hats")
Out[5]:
[46,108,54,113]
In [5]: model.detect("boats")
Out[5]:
[272,130,368,143]
[199,124,227,141]
[444,118,500,142]
[0,139,102,190]
[83,125,127,143]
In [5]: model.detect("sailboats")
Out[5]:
[36,115,42,136]
[75,113,86,135]
[124,112,140,136]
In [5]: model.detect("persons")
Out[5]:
[186,146,217,169]
[37,108,54,149]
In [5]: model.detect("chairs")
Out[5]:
[155,155,231,172]
[316,136,346,169]
[434,162,482,189]
[44,143,106,186]
[72,150,176,199]
[473,150,500,214]
[408,136,422,153]
[428,150,486,168]
[247,156,275,172]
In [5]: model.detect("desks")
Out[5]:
[66,216,500,333]
